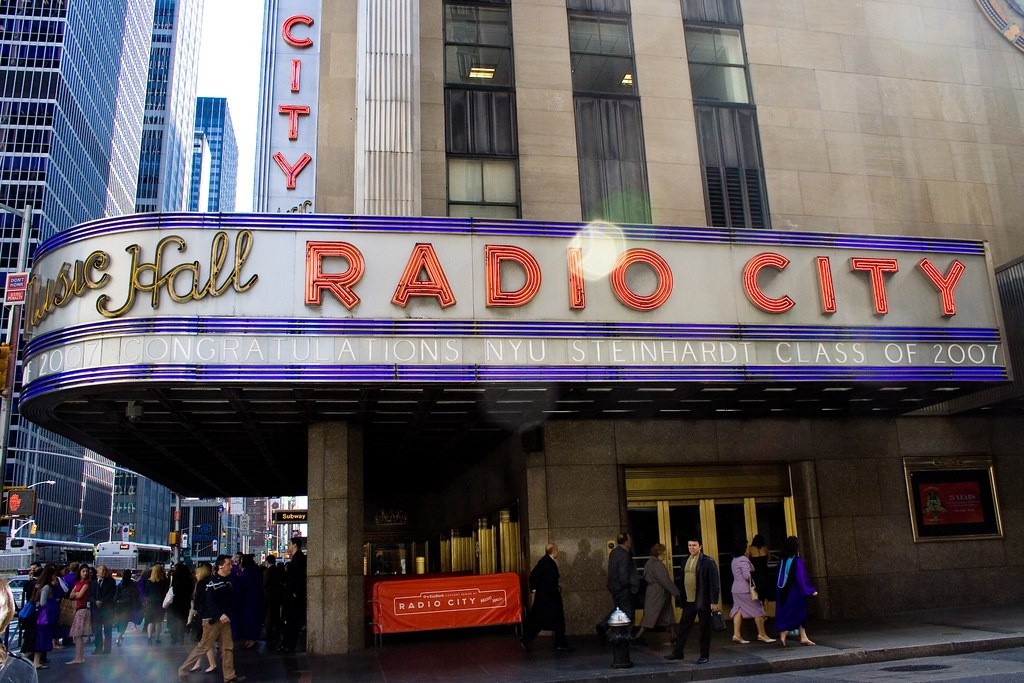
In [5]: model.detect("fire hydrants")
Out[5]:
[606,606,635,668]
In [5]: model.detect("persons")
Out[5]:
[0,537,307,683]
[595,532,818,664]
[520,543,575,653]
[927,491,941,519]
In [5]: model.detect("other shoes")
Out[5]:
[780,639,786,648]
[800,640,815,646]
[190,665,200,672]
[200,665,217,674]
[65,658,85,664]
[37,665,49,669]
[634,638,647,646]
[670,635,679,643]
[90,635,184,654]
[554,647,575,652]
[228,675,246,683]
[240,641,256,650]
[732,635,750,644]
[19,601,35,620]
[521,640,530,652]
[597,623,605,640]
[757,635,776,642]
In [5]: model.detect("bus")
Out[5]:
[5,537,94,579]
[94,540,172,585]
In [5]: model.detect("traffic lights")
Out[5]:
[7,490,36,515]
[30,525,38,534]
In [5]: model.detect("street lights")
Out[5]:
[181,525,201,547]
[11,481,56,538]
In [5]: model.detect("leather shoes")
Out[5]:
[664,654,684,660]
[697,657,708,664]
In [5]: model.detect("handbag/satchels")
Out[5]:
[35,607,48,625]
[750,576,758,600]
[162,587,174,608]
[711,608,727,633]
[58,598,77,625]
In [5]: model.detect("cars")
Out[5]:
[7,579,30,611]
